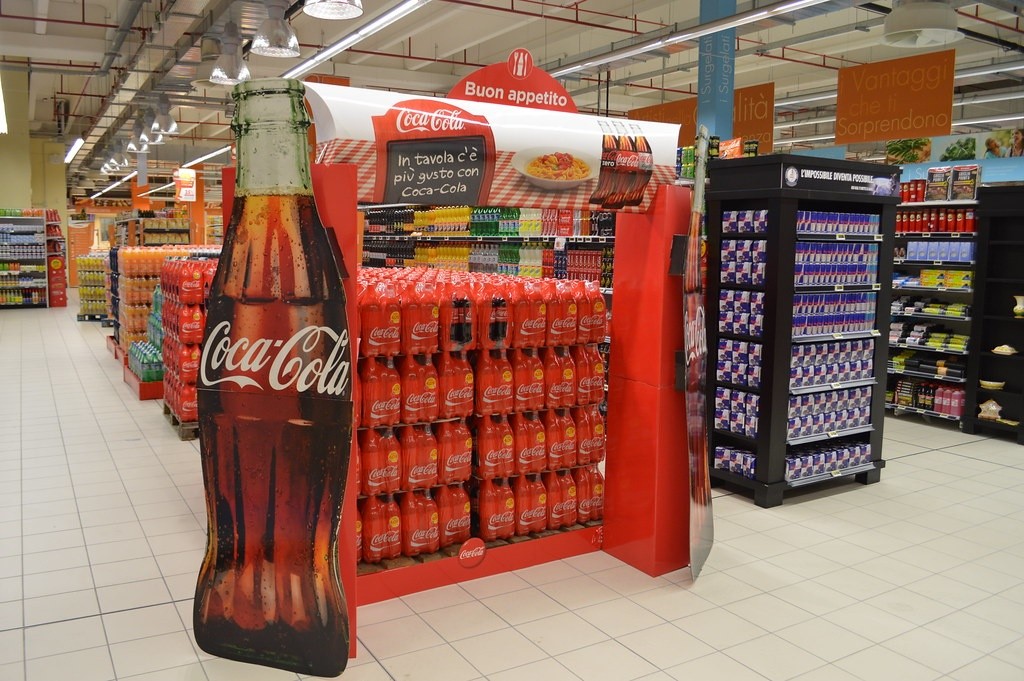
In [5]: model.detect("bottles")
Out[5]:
[193,78,353,677]
[77,202,223,423]
[1013,296,1024,318]
[0,208,65,307]
[589,121,653,210]
[911,379,966,417]
[362,205,615,293]
[685,128,709,501]
[355,260,612,565]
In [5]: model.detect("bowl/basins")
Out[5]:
[512,148,601,190]
[979,379,1005,390]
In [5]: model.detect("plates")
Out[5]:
[990,350,1018,356]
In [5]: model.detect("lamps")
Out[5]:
[101,0,364,175]
[547,0,833,78]
[774,60,1024,146]
[280,0,434,78]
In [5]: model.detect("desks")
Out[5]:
[313,138,678,212]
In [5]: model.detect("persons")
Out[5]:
[984,137,1005,159]
[1006,128,1024,156]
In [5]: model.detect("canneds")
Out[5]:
[677,146,695,178]
[895,209,975,232]
[899,179,926,202]
[0,245,45,259]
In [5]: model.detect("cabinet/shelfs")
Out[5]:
[706,154,901,509]
[108,164,222,248]
[884,185,1024,444]
[357,203,616,392]
[0,209,63,308]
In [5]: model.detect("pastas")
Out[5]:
[527,153,590,180]
